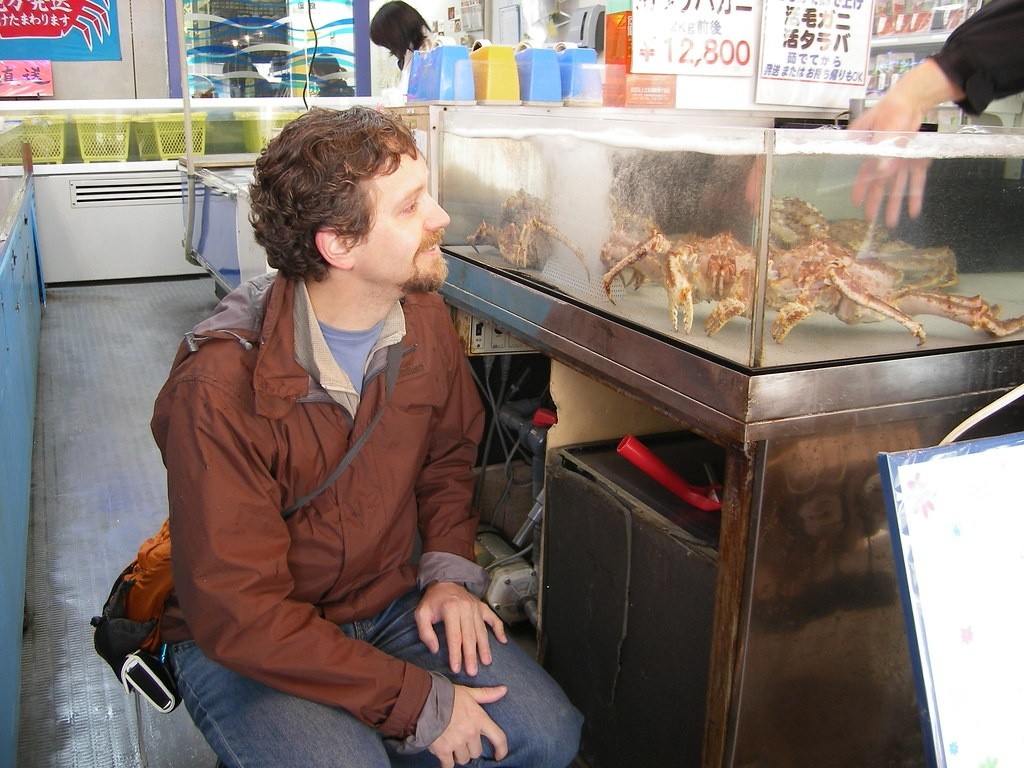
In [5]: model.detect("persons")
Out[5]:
[311,52,354,97]
[151,103,589,768]
[223,60,274,98]
[844,0,1024,145]
[369,0,468,161]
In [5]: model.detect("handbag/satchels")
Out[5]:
[91,518,182,713]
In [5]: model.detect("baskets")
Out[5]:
[234,111,303,153]
[71,113,130,163]
[0,114,67,166]
[131,113,208,161]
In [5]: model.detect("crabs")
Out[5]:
[466,188,1024,348]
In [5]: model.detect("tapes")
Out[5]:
[431,37,578,54]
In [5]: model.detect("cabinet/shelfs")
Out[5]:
[861,32,1024,129]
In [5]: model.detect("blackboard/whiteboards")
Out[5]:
[877,431,1024,768]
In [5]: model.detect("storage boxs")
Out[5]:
[0,112,302,165]
[406,44,604,108]
